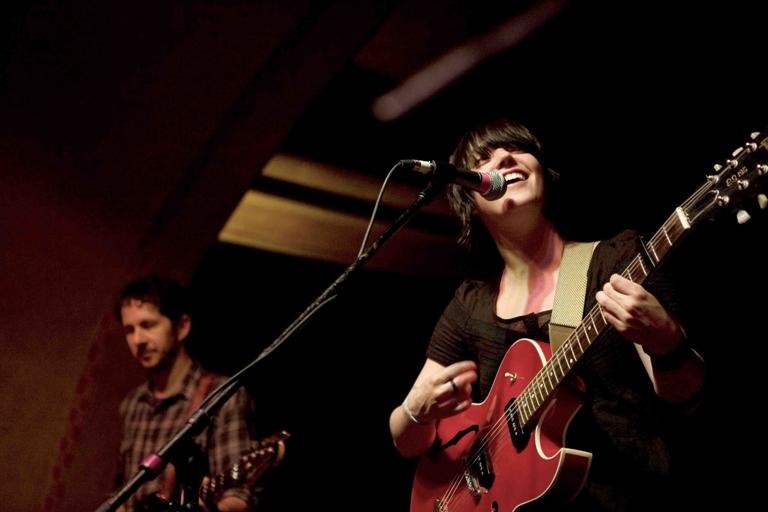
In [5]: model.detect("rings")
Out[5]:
[448,378,458,394]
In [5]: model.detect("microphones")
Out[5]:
[410,155,508,204]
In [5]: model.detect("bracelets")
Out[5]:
[641,335,693,373]
[400,398,433,427]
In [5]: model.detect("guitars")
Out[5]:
[409,130,768,512]
[132,430,286,512]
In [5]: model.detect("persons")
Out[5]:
[104,275,263,512]
[386,114,711,510]
